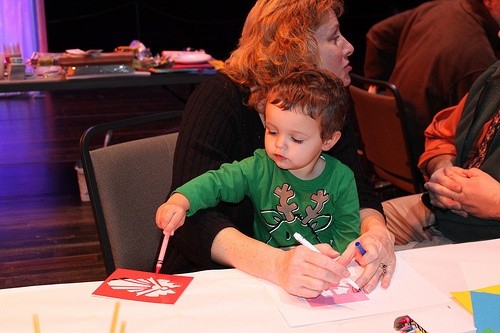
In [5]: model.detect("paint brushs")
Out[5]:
[155,233,169,279]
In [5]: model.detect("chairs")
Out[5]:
[346,73,423,195]
[78,110,184,278]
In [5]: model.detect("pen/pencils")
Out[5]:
[293,232,363,293]
[356,242,388,269]
[3,43,22,55]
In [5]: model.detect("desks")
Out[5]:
[0,51,220,94]
[0,238,500,333]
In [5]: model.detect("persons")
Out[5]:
[365,0,500,254]
[155,69,362,256]
[150,0,397,299]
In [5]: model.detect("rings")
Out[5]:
[379,262,388,273]
